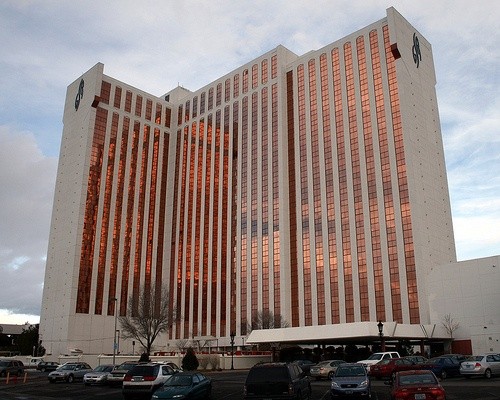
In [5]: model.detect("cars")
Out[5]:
[106,360,137,389]
[47,361,94,384]
[151,372,214,400]
[328,363,373,400]
[37,361,60,373]
[459,353,500,379]
[293,360,316,374]
[309,359,346,381]
[354,351,465,384]
[83,364,120,387]
[165,362,180,372]
[384,369,445,400]
[121,364,177,400]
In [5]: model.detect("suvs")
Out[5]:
[242,360,313,400]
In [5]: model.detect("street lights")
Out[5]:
[116,329,120,356]
[113,297,117,365]
[229,331,235,370]
[377,320,384,351]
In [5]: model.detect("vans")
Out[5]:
[0,360,25,377]
